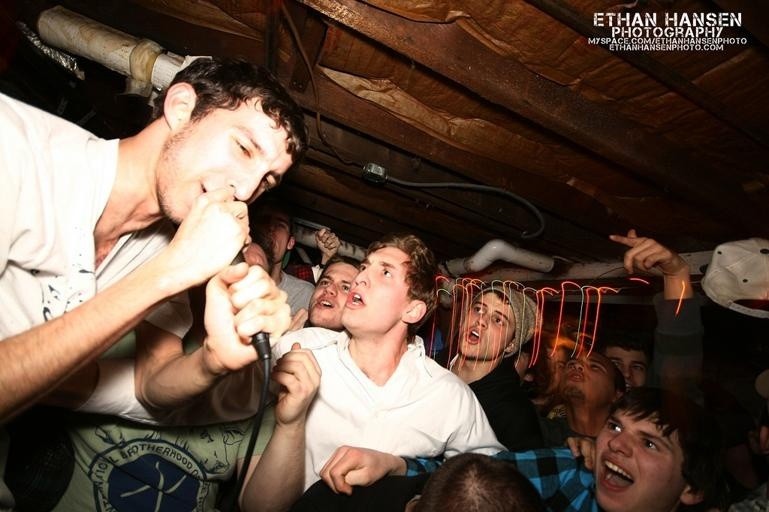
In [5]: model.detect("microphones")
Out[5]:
[224,246,272,361]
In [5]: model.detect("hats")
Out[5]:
[700,237,769,318]
[468,285,544,358]
